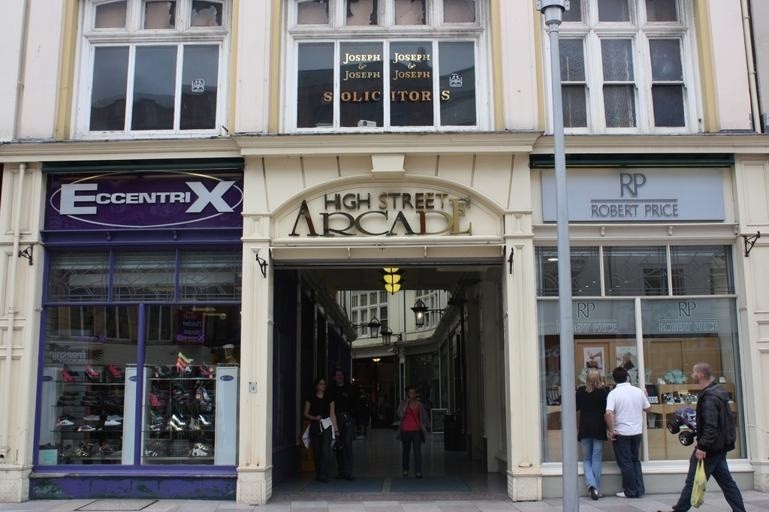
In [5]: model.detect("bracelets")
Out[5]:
[335,430,340,433]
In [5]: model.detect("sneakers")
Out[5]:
[616,491,627,498]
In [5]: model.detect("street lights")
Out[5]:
[535,0,582,512]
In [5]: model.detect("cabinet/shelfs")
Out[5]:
[144,376,216,462]
[644,382,738,417]
[575,336,725,386]
[50,382,125,461]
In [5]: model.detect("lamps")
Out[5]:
[19,239,37,266]
[507,242,525,275]
[743,229,760,258]
[353,317,382,338]
[250,246,268,279]
[410,298,449,326]
[379,267,405,296]
[379,325,403,347]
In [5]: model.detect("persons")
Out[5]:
[603,366,653,500]
[656,361,748,511]
[617,350,635,371]
[576,366,611,501]
[303,376,342,485]
[329,366,361,481]
[394,385,431,480]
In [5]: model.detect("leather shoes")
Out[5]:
[590,487,598,500]
